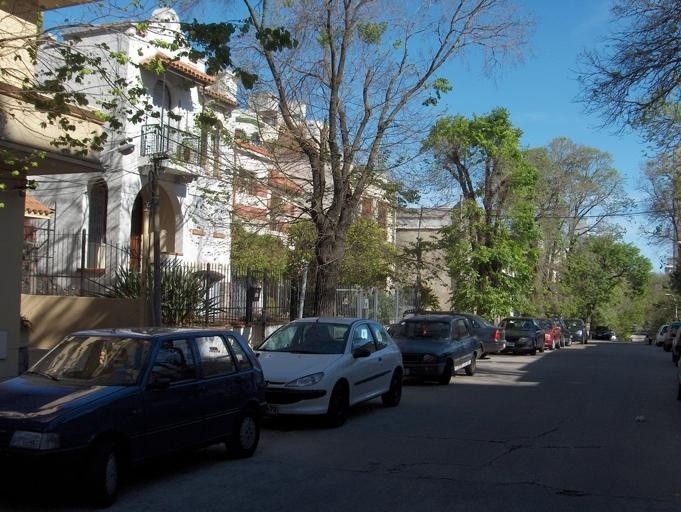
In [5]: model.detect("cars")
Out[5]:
[389,311,612,387]
[655,320,681,402]
[251,316,406,430]
[0,327,267,507]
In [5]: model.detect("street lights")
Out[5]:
[665,293,678,319]
